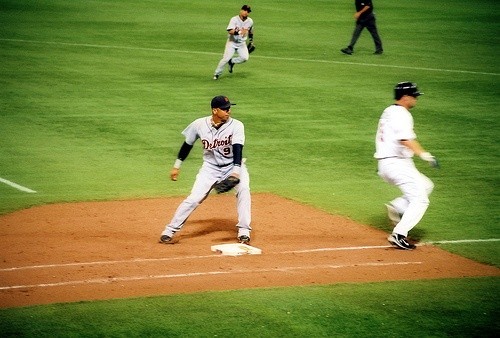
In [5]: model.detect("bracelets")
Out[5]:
[238,31,241,34]
[360,11,362,14]
[234,166,240,173]
[175,159,182,168]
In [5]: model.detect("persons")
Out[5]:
[341,0,383,55]
[160,96,251,242]
[374,81,437,251]
[213,5,255,80]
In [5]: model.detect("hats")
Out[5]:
[211,96,236,109]
[242,5,251,12]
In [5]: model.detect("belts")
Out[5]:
[216,163,231,168]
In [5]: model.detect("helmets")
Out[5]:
[394,82,424,99]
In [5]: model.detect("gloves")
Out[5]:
[419,152,438,167]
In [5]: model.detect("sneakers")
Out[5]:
[228,59,233,72]
[214,76,218,80]
[341,47,352,54]
[160,235,172,243]
[388,233,415,249]
[239,235,249,243]
[374,49,382,54]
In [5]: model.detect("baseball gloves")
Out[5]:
[214,176,240,194]
[247,42,256,54]
